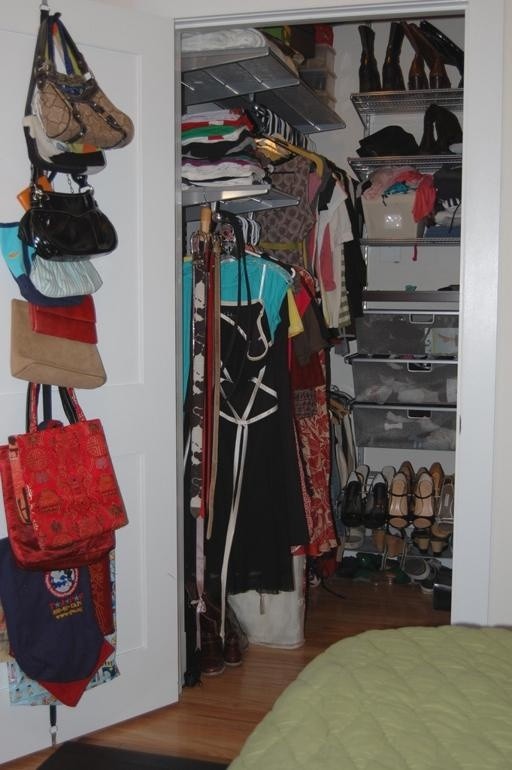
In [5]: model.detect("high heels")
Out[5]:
[385,524,409,558]
[340,471,363,527]
[412,471,436,529]
[400,461,415,491]
[431,537,448,556]
[343,524,365,549]
[436,476,454,534]
[373,529,385,554]
[361,471,388,529]
[387,471,409,528]
[411,529,430,555]
[412,467,429,504]
[428,462,444,497]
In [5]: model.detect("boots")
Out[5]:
[399,20,429,90]
[382,21,408,91]
[418,105,461,153]
[358,25,381,92]
[420,19,464,88]
[408,22,451,89]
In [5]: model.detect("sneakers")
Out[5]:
[397,556,437,584]
[419,559,440,593]
[307,566,321,588]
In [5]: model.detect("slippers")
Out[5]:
[355,464,370,498]
[381,465,395,491]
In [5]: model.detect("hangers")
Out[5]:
[251,102,323,178]
[213,210,297,285]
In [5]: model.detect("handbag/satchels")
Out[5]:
[0,537,100,681]
[29,295,98,345]
[16,166,119,262]
[0,221,82,306]
[23,15,135,175]
[88,555,115,636]
[0,381,116,570]
[29,252,103,297]
[8,382,129,549]
[10,298,107,389]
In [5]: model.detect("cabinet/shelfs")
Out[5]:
[173,21,347,414]
[344,86,463,574]
